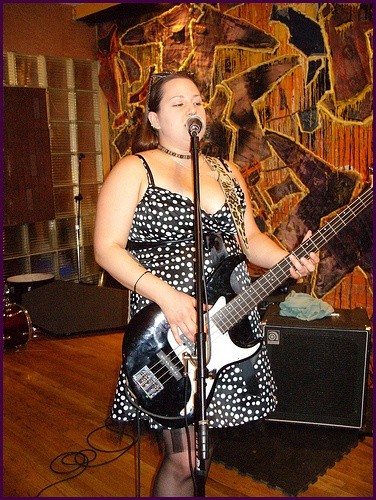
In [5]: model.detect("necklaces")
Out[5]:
[157,144,202,159]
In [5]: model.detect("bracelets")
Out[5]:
[134,271,150,294]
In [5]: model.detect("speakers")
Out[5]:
[259,302,371,432]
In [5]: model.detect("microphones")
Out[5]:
[186,115,204,134]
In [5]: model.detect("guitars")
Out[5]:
[121,186,376,431]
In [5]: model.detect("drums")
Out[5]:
[6,272,55,305]
[2,303,32,350]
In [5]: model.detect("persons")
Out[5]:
[93,70,320,497]
